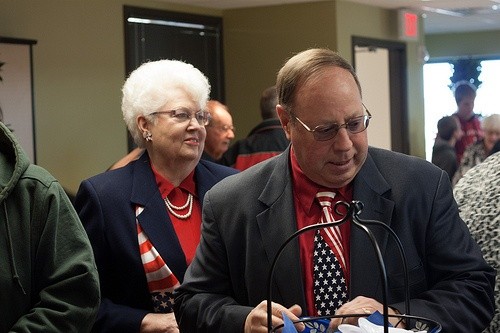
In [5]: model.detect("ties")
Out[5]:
[313,188,351,320]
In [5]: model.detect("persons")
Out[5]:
[75,59,243,333]
[432,81,500,333]
[174,47,497,333]
[0,121,102,333]
[106,86,291,178]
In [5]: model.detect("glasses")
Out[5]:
[223,124,234,130]
[148,110,212,125]
[291,103,372,141]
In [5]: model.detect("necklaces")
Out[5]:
[166,193,191,210]
[163,196,193,219]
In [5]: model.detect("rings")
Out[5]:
[363,308,370,313]
